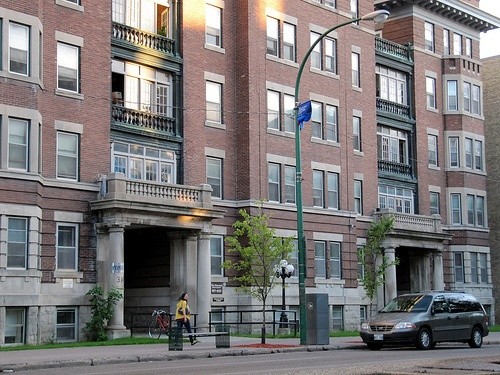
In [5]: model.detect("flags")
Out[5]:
[297,99,312,131]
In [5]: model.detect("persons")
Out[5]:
[175,293,198,345]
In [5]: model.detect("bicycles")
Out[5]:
[148,309,184,340]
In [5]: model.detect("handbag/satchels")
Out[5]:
[178,300,189,315]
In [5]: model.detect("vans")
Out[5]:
[358,292,490,350]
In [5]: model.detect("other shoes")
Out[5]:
[191,340,198,345]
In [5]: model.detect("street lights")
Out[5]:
[272,259,294,334]
[292,9,391,347]
